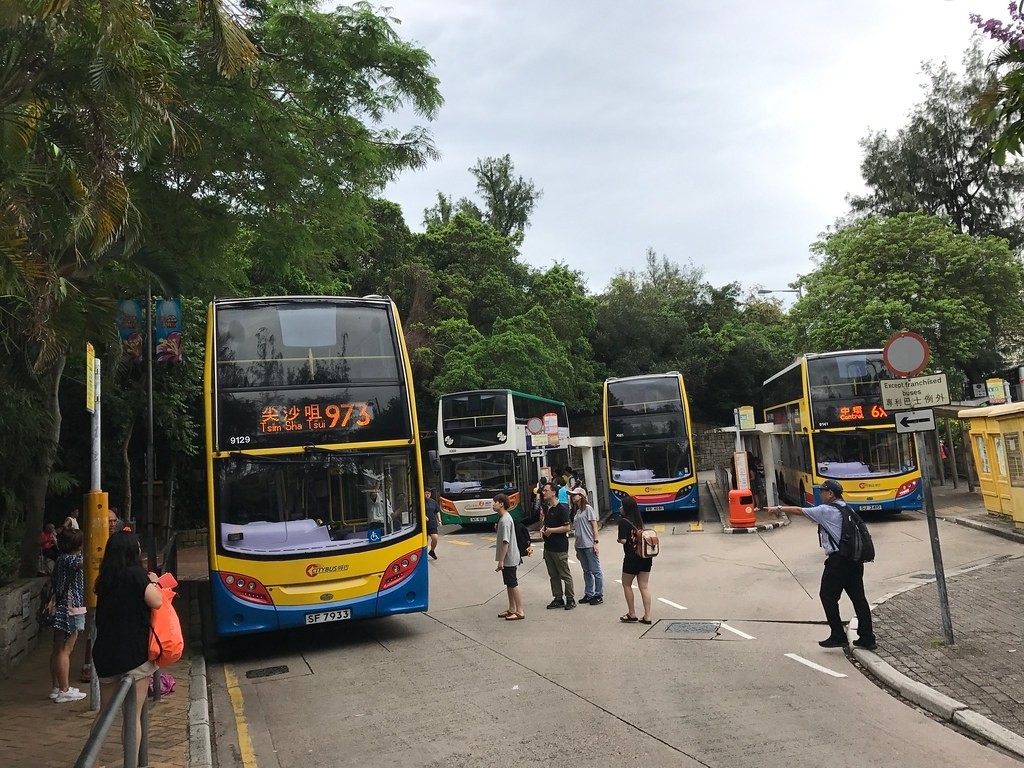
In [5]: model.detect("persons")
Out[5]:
[565,487,604,605]
[729,450,765,510]
[539,482,576,610]
[365,477,407,534]
[424,487,442,559]
[493,494,526,622]
[763,479,876,650]
[40,505,122,565]
[617,496,652,624]
[91,531,163,768]
[536,466,583,537]
[48,527,85,702]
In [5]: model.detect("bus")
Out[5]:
[602,370,698,516]
[435,389,573,529]
[762,347,923,517]
[205,294,428,640]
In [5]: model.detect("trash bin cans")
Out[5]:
[728,489,756,528]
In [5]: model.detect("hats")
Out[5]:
[540,477,547,484]
[566,488,587,497]
[812,479,843,494]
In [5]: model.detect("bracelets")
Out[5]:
[776,505,782,512]
[594,540,598,543]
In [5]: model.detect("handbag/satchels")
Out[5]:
[148,586,184,669]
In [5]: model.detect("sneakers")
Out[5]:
[49,687,79,699]
[55,687,87,703]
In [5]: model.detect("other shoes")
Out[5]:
[590,596,603,605]
[547,598,565,608]
[579,594,592,603]
[429,551,437,560]
[819,631,848,647]
[565,599,576,610]
[853,634,876,649]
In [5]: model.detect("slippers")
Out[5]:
[639,617,651,624]
[506,613,525,620]
[619,614,638,621]
[498,610,513,617]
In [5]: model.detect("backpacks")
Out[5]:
[514,517,531,557]
[37,558,75,626]
[821,503,874,562]
[623,518,659,558]
